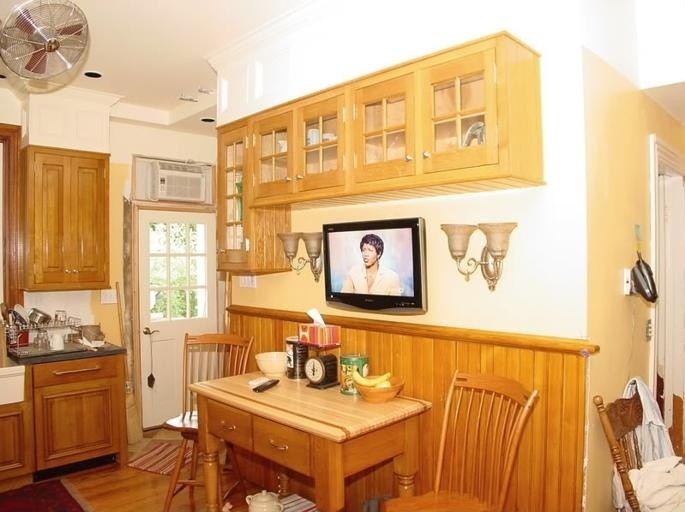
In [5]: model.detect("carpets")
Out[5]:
[1,480,86,512]
[130,437,195,477]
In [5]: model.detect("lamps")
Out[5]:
[278,233,325,281]
[439,221,518,292]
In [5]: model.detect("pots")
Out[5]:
[26,309,51,329]
[245,490,284,512]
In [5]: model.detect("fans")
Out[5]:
[1,0,90,79]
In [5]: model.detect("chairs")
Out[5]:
[383,371,540,511]
[160,333,256,512]
[593,386,685,512]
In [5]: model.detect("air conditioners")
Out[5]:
[152,161,207,203]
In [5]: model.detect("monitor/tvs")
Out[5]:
[322,217,427,313]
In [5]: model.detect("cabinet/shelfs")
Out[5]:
[252,83,349,210]
[214,117,289,275]
[20,145,113,292]
[32,354,129,477]
[0,404,34,488]
[349,29,545,197]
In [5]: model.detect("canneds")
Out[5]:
[340,354,369,395]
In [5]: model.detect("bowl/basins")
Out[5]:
[255,351,288,378]
[354,376,405,403]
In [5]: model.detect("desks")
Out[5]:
[189,372,433,512]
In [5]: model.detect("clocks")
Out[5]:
[303,354,341,390]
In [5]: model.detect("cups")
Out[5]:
[33,331,64,351]
[55,309,67,323]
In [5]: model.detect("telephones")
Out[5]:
[632,260,658,302]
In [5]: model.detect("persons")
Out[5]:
[340,235,401,296]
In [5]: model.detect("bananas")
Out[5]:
[352,371,391,387]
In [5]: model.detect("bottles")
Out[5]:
[7,312,16,347]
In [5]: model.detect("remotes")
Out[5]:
[254,379,281,392]
